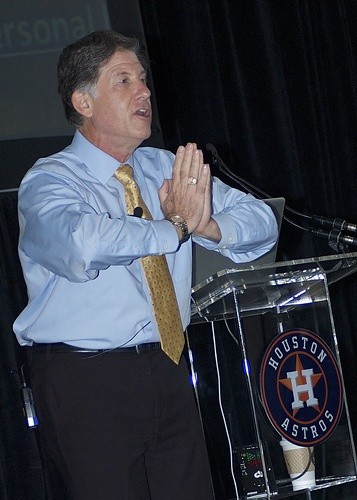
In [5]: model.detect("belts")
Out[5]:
[25,335,162,354]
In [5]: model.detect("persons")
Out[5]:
[11,28,279,500]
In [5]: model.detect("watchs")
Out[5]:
[165,213,189,240]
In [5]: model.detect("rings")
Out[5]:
[187,176,198,187]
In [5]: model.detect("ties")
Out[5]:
[114,163,186,364]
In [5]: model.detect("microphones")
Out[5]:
[213,160,357,248]
[130,207,143,217]
[212,151,357,233]
